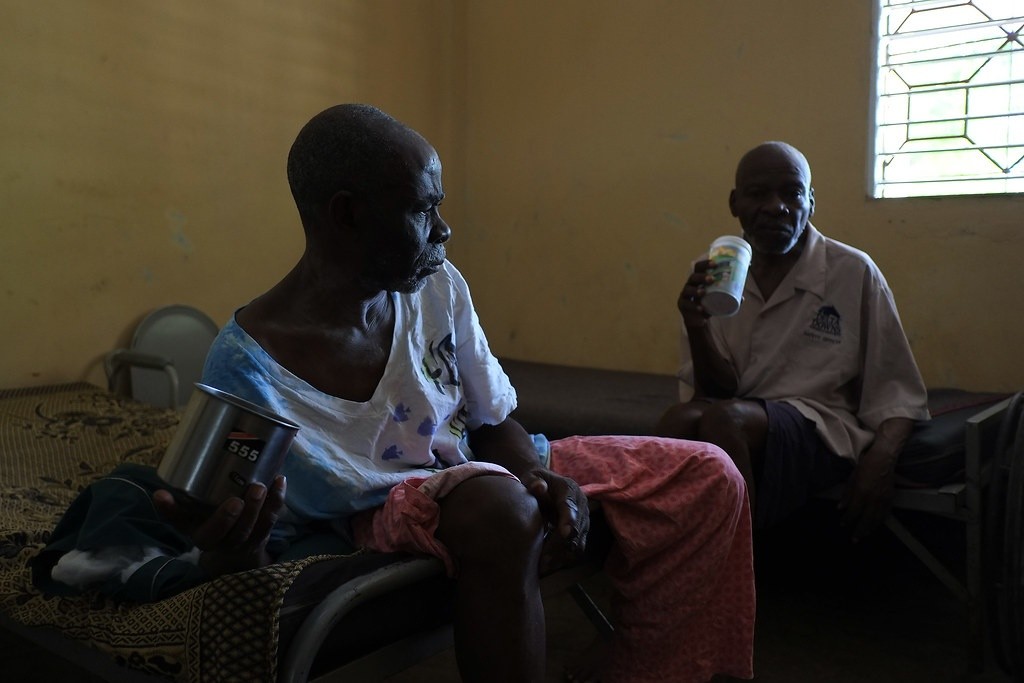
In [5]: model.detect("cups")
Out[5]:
[156,380,300,519]
[701,235,752,318]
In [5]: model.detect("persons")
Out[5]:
[151,105,757,683]
[656,140,932,551]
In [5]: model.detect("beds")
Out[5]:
[497,355,1024,674]
[0,380,627,683]
